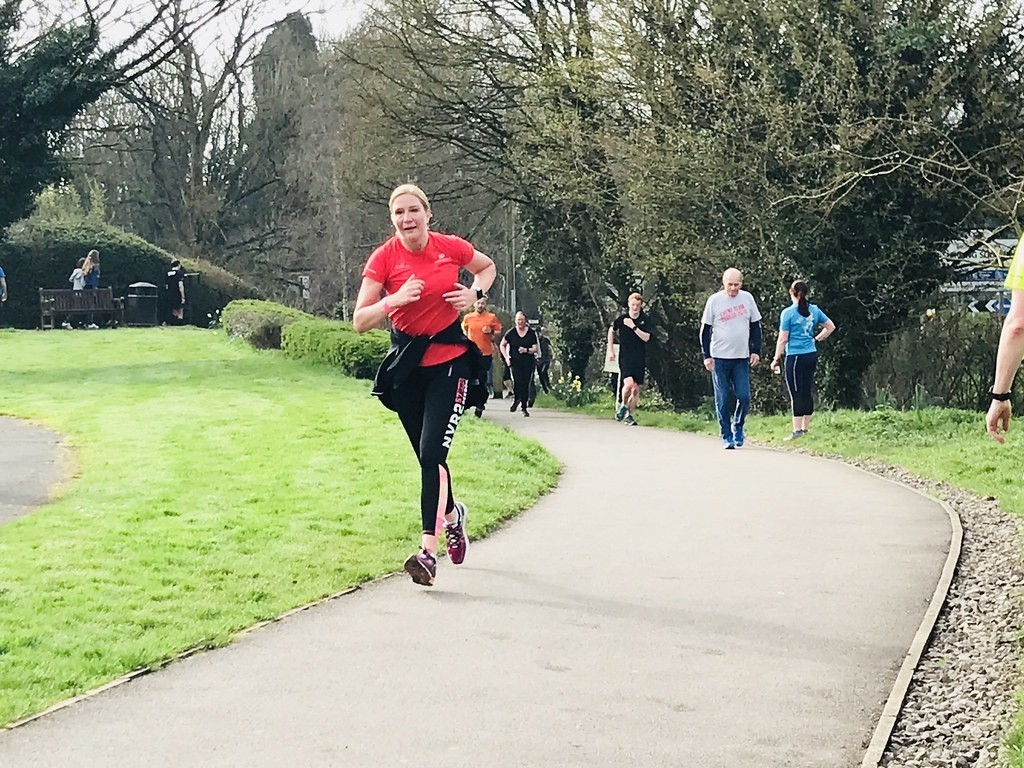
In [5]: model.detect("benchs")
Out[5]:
[38,285,125,331]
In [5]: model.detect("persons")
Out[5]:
[699,267,762,449]
[986,232,1024,444]
[0,267,8,301]
[770,280,836,442]
[165,259,185,326]
[62,250,101,329]
[462,294,503,418]
[607,292,657,425]
[486,311,551,418]
[352,184,496,586]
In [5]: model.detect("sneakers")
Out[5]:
[783,429,808,441]
[731,423,744,447]
[61,322,73,330]
[722,436,735,448]
[403,546,437,586]
[615,404,628,421]
[624,416,638,426]
[444,501,470,566]
[88,323,99,329]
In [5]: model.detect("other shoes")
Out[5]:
[521,406,530,417]
[473,406,485,419]
[510,399,521,411]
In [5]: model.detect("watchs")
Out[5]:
[988,386,1011,401]
[473,288,483,300]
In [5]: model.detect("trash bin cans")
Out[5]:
[127,282,156,326]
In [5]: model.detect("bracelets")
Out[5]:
[383,297,396,312]
[633,326,638,330]
[773,357,779,361]
[814,337,818,343]
